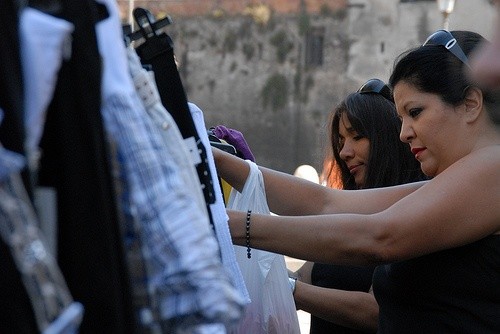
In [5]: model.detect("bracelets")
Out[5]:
[288,276,296,293]
[246,209,252,258]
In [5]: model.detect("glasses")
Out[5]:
[421,30,472,72]
[357,79,394,102]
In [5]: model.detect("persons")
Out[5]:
[210,29,500,334]
[286,78,428,334]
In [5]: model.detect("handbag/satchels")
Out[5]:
[218,158,297,333]
